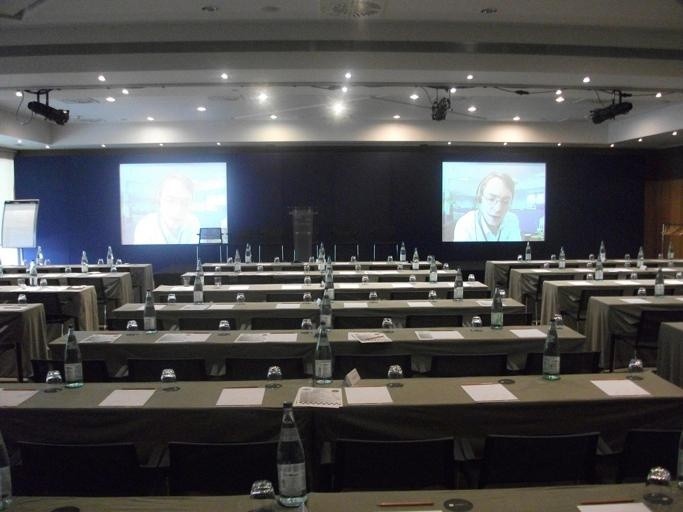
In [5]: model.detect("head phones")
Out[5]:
[474,174,503,204]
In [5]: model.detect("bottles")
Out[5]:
[0,429,13,512]
[143,288,157,332]
[542,318,561,381]
[318,287,334,329]
[677,430,683,491]
[490,285,505,329]
[313,322,334,385]
[453,267,464,301]
[64,324,84,389]
[193,269,204,305]
[276,402,309,508]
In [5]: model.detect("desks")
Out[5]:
[589,296,681,366]
[149,283,491,298]
[0,481,682,512]
[202,260,444,273]
[540,278,681,326]
[0,285,100,331]
[658,321,681,386]
[1,257,154,304]
[0,303,49,378]
[485,258,682,294]
[1,273,135,311]
[110,298,527,329]
[1,372,682,467]
[180,270,461,282]
[507,266,682,307]
[46,326,589,376]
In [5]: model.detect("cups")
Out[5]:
[627,359,643,381]
[299,318,313,334]
[0,244,123,304]
[388,364,403,388]
[126,320,138,336]
[266,366,282,389]
[552,314,563,329]
[248,479,277,512]
[303,292,312,304]
[181,242,476,286]
[45,370,62,393]
[160,368,177,391]
[470,316,484,332]
[428,289,438,301]
[217,319,231,336]
[167,293,177,304]
[641,467,673,506]
[517,240,683,297]
[368,291,378,302]
[380,317,394,333]
[236,292,246,305]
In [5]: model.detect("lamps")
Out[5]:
[589,88,633,124]
[27,87,70,126]
[430,86,451,121]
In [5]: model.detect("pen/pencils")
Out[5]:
[590,377,627,380]
[460,382,491,386]
[223,385,259,389]
[376,501,435,506]
[3,387,37,391]
[578,498,634,506]
[427,329,454,331]
[366,335,384,341]
[120,387,158,390]
[351,384,385,387]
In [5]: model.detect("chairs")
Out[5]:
[1,291,79,341]
[536,273,661,303]
[2,430,682,494]
[158,290,491,303]
[189,275,459,284]
[3,267,143,303]
[575,289,682,332]
[105,313,536,330]
[1,320,24,382]
[510,262,683,269]
[30,349,607,384]
[1,277,108,330]
[634,311,680,370]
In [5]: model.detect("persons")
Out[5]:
[134,176,206,245]
[454,172,521,242]
